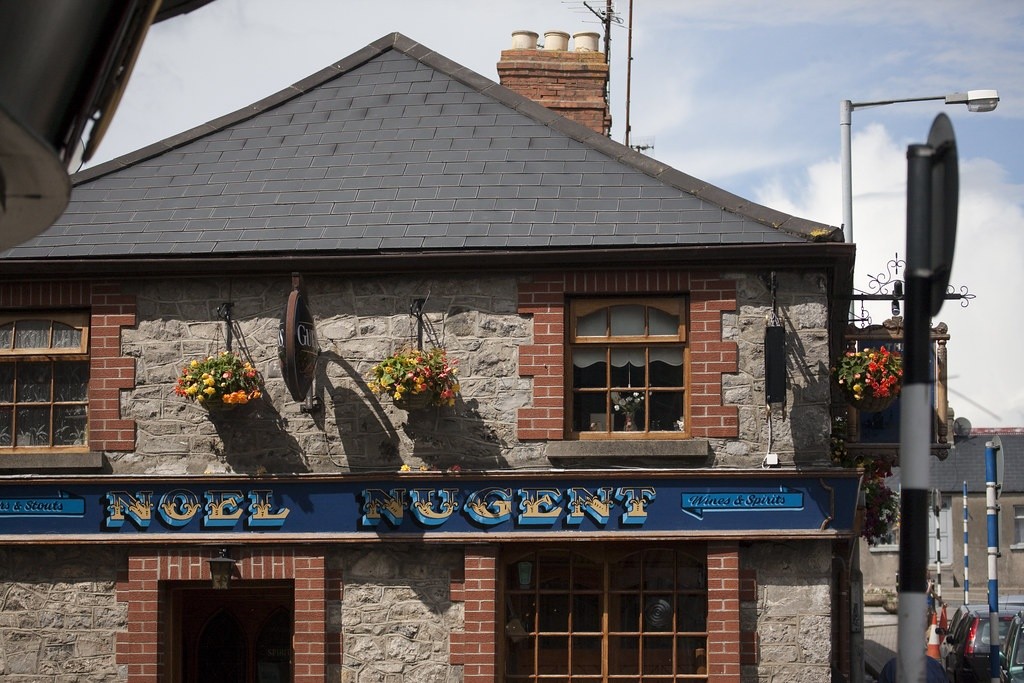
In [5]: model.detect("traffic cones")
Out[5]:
[938,603,947,644]
[926,611,941,659]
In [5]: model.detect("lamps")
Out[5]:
[206,546,238,590]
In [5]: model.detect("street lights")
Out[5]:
[840,89,1000,324]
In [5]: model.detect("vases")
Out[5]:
[513,31,539,49]
[199,399,238,412]
[392,394,432,410]
[544,31,570,51]
[572,32,600,52]
[846,386,896,412]
[622,414,637,432]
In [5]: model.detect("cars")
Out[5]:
[945,610,1021,683]
[935,605,1024,669]
[999,610,1024,683]
[997,595,1024,605]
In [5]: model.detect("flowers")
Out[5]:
[613,383,652,419]
[830,346,903,400]
[175,351,262,405]
[367,347,460,407]
